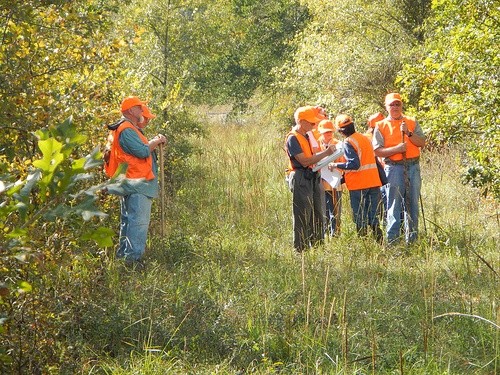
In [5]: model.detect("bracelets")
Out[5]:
[409,132,413,137]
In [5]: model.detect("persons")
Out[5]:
[104,96,169,266]
[371,93,426,248]
[285,105,405,255]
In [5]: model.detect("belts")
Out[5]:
[392,160,418,165]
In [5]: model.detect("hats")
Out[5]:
[317,121,335,133]
[368,112,385,127]
[121,95,145,111]
[138,101,156,118]
[309,106,327,119]
[385,92,403,105]
[335,113,351,127]
[294,106,320,123]
